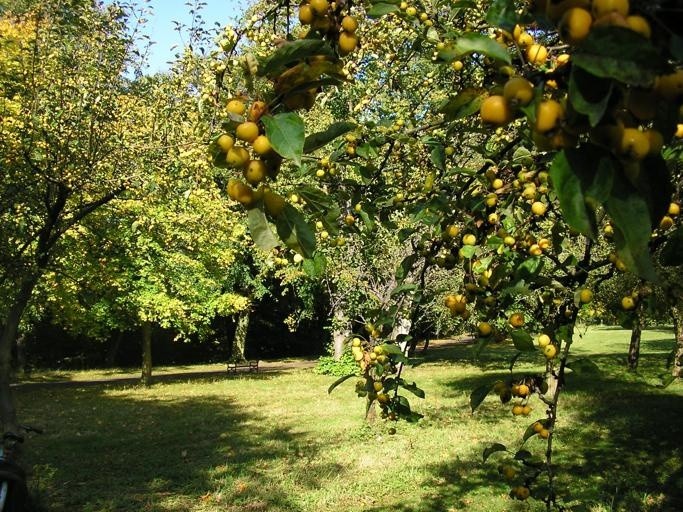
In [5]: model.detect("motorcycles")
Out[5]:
[0,418,43,512]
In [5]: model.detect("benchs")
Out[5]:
[225,358,260,376]
